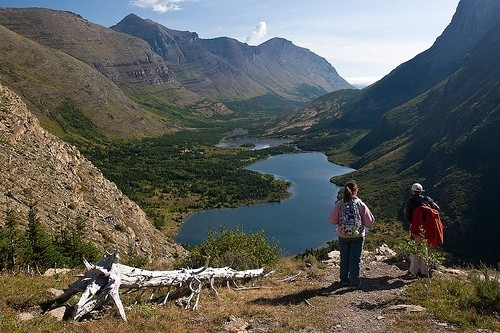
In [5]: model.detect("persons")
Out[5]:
[403,183,441,278]
[328,181,375,289]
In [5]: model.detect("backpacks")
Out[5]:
[411,195,443,249]
[340,198,363,237]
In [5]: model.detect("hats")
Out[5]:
[410,183,423,192]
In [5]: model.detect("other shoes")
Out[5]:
[416,271,430,278]
[400,270,419,280]
